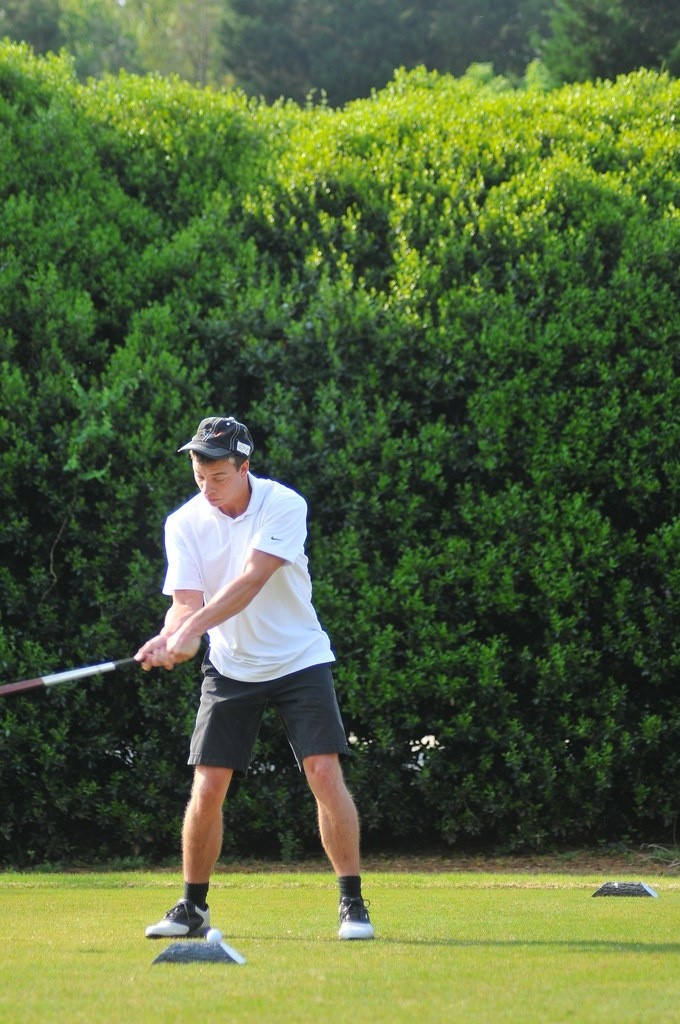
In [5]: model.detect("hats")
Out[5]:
[176,414,254,459]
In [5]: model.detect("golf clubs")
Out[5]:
[0,657,138,696]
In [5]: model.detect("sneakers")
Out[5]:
[337,896,374,940]
[145,897,210,936]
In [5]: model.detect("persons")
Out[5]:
[135,416,375,941]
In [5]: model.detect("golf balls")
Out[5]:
[206,929,222,943]
